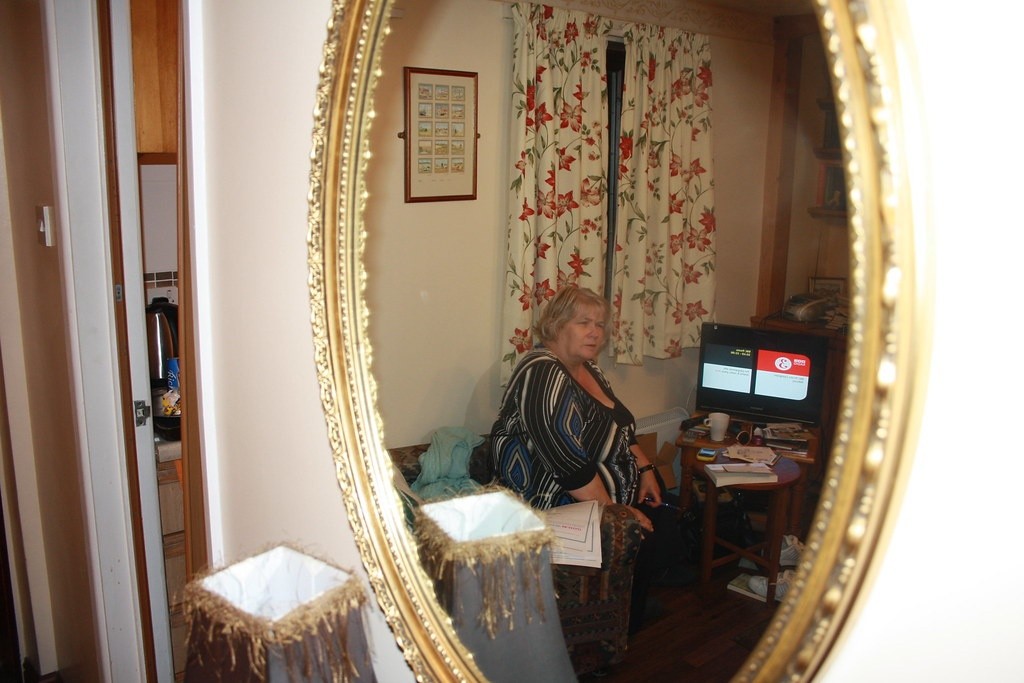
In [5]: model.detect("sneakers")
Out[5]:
[748,569,797,602]
[759,534,806,567]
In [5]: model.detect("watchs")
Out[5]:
[637,464,656,476]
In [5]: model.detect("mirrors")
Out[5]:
[303,3,931,682]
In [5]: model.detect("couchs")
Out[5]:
[387,432,649,679]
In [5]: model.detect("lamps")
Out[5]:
[179,546,379,683]
[407,492,582,683]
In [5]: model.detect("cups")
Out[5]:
[703,412,730,442]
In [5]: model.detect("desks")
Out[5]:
[673,411,823,544]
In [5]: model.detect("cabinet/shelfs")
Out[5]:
[749,11,851,464]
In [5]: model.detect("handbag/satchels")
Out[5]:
[468,441,496,485]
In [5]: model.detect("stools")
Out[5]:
[691,446,799,604]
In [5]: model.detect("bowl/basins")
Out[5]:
[152,395,182,442]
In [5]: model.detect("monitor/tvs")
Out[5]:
[697,322,828,425]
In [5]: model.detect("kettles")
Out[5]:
[145,297,180,389]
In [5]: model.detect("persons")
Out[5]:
[489,286,662,679]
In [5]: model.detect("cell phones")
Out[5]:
[697,447,718,462]
